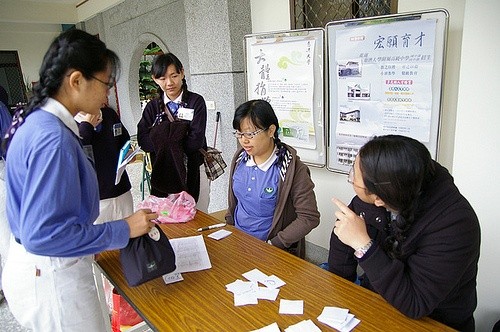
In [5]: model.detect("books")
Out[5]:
[117,140,132,174]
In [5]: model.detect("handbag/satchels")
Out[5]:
[134,190,197,224]
[119,221,177,287]
[203,146,227,181]
[110,287,144,332]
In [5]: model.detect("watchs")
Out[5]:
[354,239,373,258]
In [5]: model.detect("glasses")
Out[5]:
[347,167,367,190]
[232,127,266,139]
[92,76,116,91]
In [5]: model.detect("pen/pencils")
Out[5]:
[197,223,227,231]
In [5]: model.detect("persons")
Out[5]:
[0,28,158,331]
[224,99,321,260]
[0,100,14,303]
[135,52,207,204]
[74,106,133,310]
[328,134,481,331]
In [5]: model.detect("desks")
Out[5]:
[92,206,458,332]
